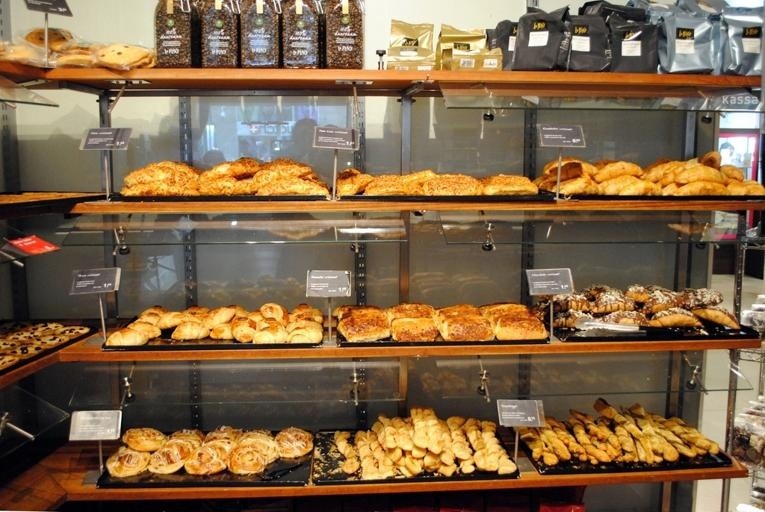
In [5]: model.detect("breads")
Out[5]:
[0,322,91,372]
[106,302,324,347]
[514,396,720,468]
[336,167,539,197]
[534,149,762,195]
[334,407,518,480]
[106,427,315,478]
[323,303,549,343]
[119,157,330,196]
[534,284,741,330]
[0,25,154,71]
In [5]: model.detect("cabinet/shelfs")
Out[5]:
[0,61,765,512]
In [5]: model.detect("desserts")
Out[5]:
[154,213,706,311]
[135,357,680,419]
